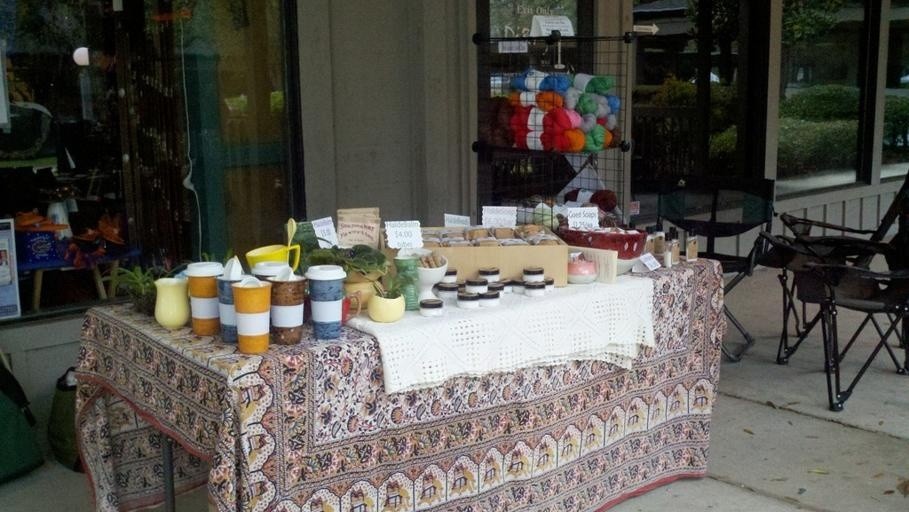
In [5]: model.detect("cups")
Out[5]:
[245,243,301,274]
[213,274,248,342]
[231,278,274,355]
[153,276,190,331]
[264,274,305,345]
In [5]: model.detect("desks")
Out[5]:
[77,258,725,512]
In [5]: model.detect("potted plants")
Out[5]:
[95,259,194,314]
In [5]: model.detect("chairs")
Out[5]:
[653,164,908,412]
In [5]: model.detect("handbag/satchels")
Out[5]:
[48,365,86,472]
[0,353,45,485]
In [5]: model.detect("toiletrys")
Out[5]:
[417,264,556,318]
[628,222,700,270]
[566,252,602,285]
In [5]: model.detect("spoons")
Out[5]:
[286,218,297,262]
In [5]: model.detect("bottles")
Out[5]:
[306,264,345,342]
[185,260,222,337]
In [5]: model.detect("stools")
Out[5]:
[12,257,110,313]
